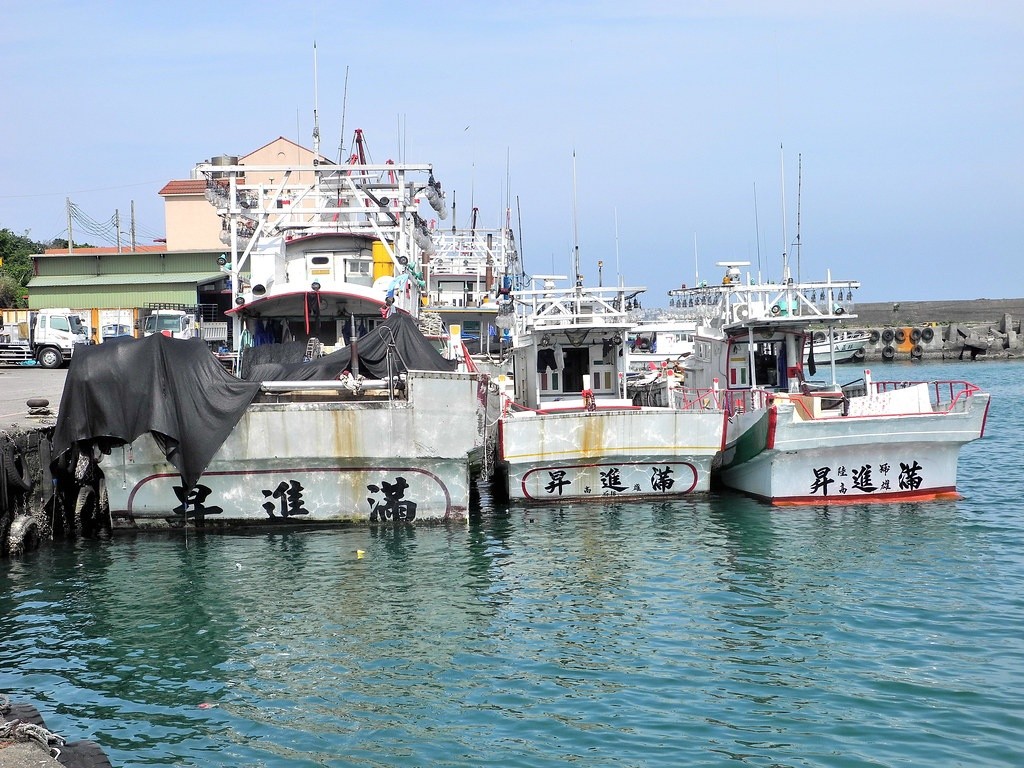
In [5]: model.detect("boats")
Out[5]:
[485,140,734,518]
[47,31,513,533]
[621,309,873,382]
[691,141,998,509]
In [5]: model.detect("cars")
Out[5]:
[101,323,130,343]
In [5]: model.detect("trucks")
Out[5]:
[0,306,89,368]
[133,303,229,359]
